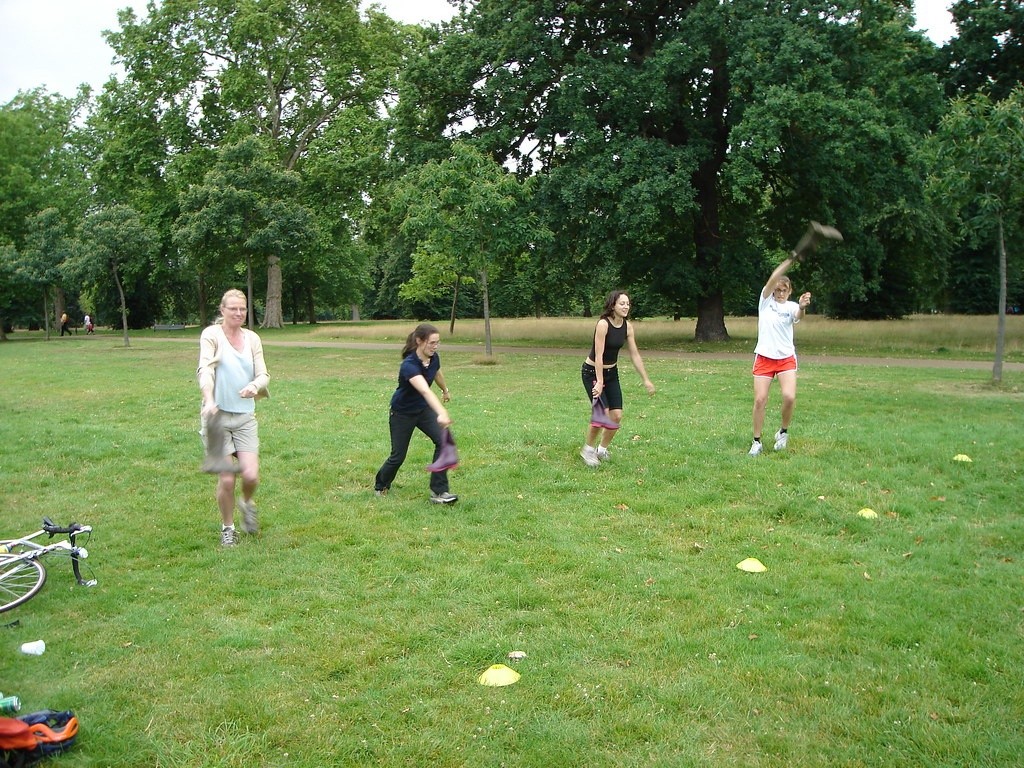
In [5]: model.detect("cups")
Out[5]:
[21,640,45,655]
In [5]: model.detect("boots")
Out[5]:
[791,220,843,266]
[202,411,244,473]
[427,430,458,472]
[591,382,622,429]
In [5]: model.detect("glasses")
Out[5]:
[222,306,248,311]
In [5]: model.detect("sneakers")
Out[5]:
[774,429,789,452]
[431,492,458,503]
[238,496,259,536]
[580,447,601,466]
[221,527,238,548]
[748,440,763,456]
[594,448,611,461]
[376,489,388,498]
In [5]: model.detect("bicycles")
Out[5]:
[0,515,98,615]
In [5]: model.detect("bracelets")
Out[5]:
[799,307,804,310]
[442,388,449,393]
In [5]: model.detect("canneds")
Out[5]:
[0,691,21,713]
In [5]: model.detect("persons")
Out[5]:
[376,324,459,502]
[60,311,72,336]
[199,288,271,546]
[86,322,95,335]
[750,251,811,454]
[172,322,175,326]
[84,313,92,329]
[580,291,655,467]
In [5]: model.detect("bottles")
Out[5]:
[0,544,16,554]
